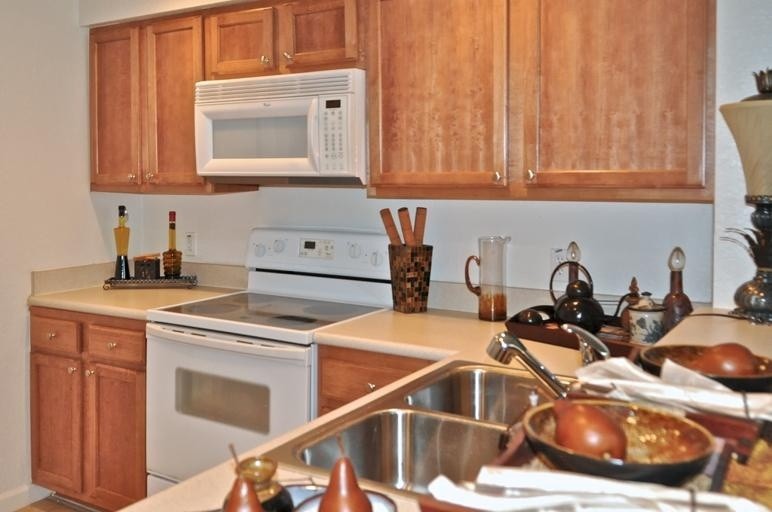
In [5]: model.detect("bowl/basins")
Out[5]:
[521,399,718,489]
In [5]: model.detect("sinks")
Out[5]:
[402,360,580,430]
[258,404,509,501]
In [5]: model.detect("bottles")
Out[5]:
[113,205,131,280]
[611,277,642,334]
[223,455,294,512]
[161,209,183,280]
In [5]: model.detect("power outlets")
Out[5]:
[550,248,568,281]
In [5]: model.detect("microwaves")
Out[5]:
[192,68,372,187]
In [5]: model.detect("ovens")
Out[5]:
[144,322,317,501]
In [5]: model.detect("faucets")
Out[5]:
[485,331,567,399]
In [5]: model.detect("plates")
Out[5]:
[640,341,772,390]
[284,484,396,511]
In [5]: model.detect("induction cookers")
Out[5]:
[142,288,387,349]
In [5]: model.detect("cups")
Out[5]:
[388,242,431,315]
[134,258,160,280]
[627,291,669,343]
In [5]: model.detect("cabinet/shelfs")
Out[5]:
[27,302,149,510]
[366,0,717,201]
[205,0,363,76]
[88,9,259,197]
[315,345,440,428]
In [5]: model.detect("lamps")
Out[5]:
[717,65,772,322]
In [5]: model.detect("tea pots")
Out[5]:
[466,232,513,323]
[548,260,604,338]
